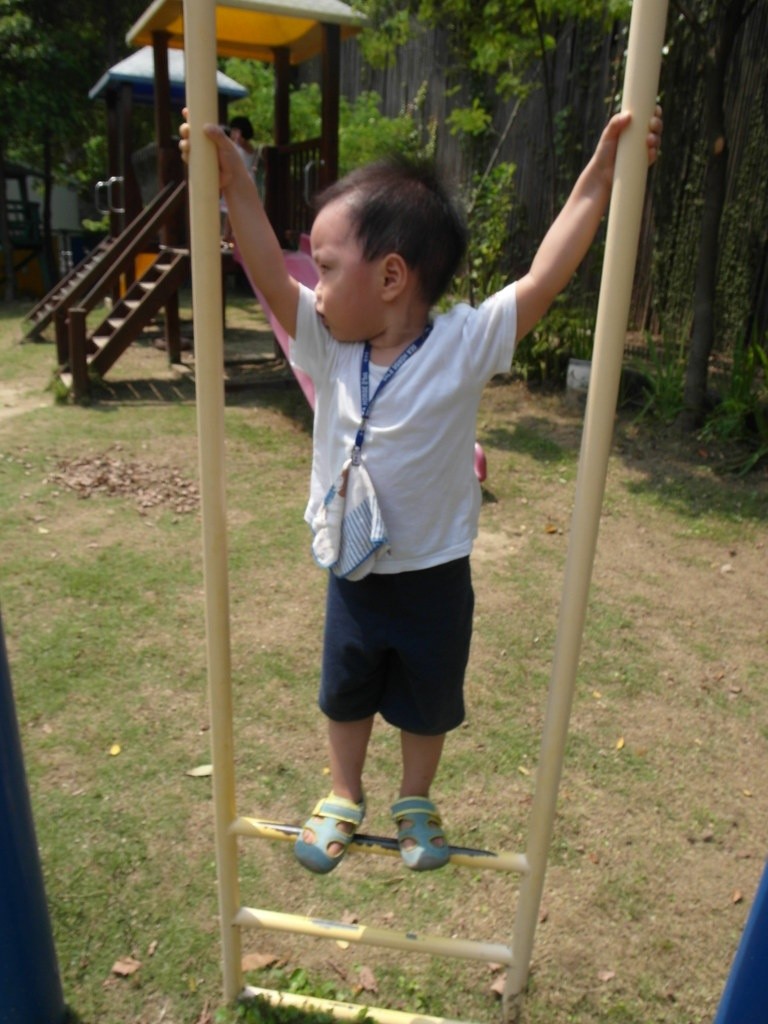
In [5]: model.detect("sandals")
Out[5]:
[294,793,368,874]
[391,796,450,870]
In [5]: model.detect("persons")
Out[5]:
[220,116,257,250]
[179,105,662,873]
